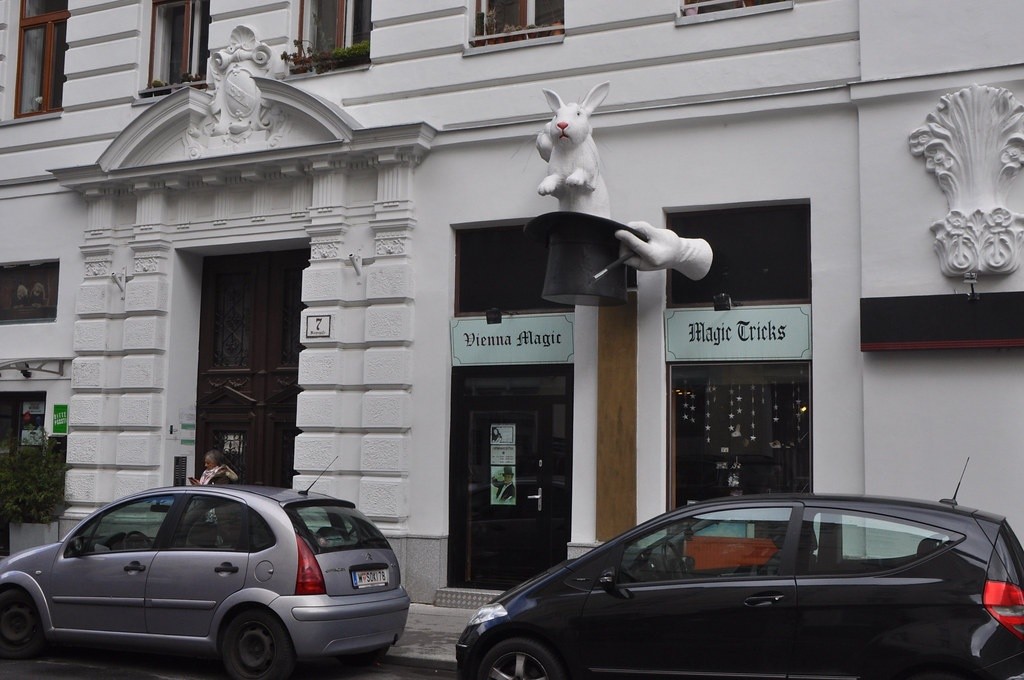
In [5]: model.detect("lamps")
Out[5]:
[485,307,520,324]
[713,293,738,312]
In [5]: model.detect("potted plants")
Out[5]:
[141,83,154,98]
[330,40,370,69]
[484,7,564,45]
[151,80,171,96]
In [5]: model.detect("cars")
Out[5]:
[0,483,412,680]
[454,494,1024,680]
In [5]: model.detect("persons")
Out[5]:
[188,449,239,547]
[22,412,39,430]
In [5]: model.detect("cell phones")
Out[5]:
[188,477,194,483]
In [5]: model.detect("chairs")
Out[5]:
[316,526,346,537]
[189,522,218,547]
[801,524,818,570]
[916,538,937,559]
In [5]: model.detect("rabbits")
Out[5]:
[537,80,610,220]
